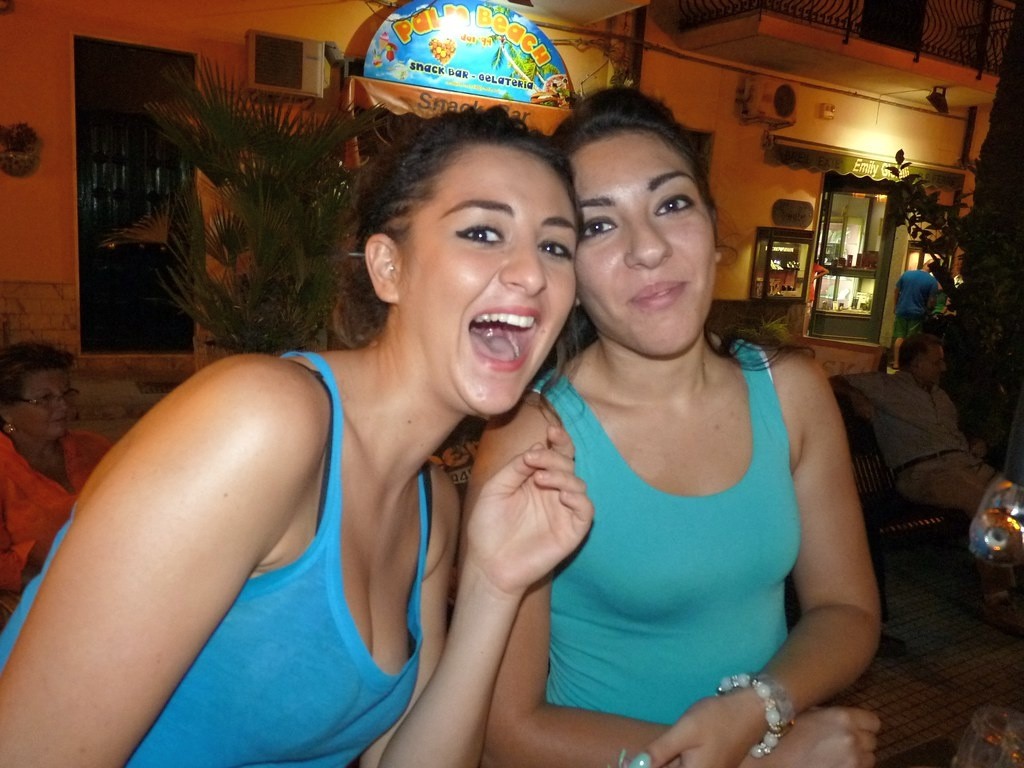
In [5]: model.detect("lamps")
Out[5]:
[926,86,948,113]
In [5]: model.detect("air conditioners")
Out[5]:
[244,28,326,101]
[743,75,798,125]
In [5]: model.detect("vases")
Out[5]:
[0,152,34,176]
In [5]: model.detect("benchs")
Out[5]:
[827,372,970,622]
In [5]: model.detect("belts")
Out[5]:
[892,448,964,475]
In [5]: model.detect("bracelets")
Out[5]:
[715,671,795,758]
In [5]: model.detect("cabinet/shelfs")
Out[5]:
[749,226,814,337]
[809,191,890,341]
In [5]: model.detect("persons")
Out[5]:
[0,107,594,767]
[459,88,882,768]
[827,333,1024,517]
[891,263,951,370]
[0,341,114,630]
[803,257,829,336]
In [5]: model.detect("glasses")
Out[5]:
[11,388,79,407]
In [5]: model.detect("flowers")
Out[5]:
[0,121,38,151]
